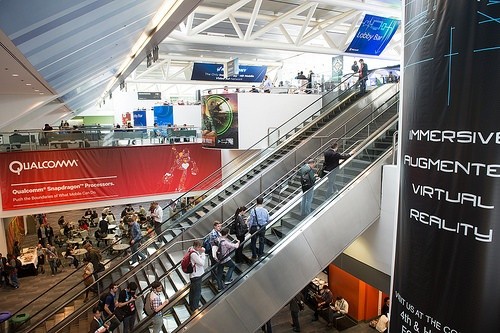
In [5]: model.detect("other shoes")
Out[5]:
[92,291,99,297]
[130,262,139,267]
[42,271,45,274]
[252,255,257,259]
[14,286,17,288]
[140,257,146,262]
[218,288,224,292]
[213,279,217,283]
[224,282,232,286]
[83,298,89,302]
[52,273,54,276]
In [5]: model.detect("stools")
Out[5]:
[62,252,70,265]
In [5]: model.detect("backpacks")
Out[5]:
[302,169,313,188]
[97,292,113,312]
[217,239,230,260]
[203,233,214,254]
[182,248,199,273]
[143,292,154,316]
[12,258,21,269]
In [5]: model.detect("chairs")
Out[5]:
[40,132,47,146]
[149,131,162,144]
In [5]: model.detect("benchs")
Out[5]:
[47,134,100,149]
[169,130,197,142]
[10,134,37,150]
[110,132,144,146]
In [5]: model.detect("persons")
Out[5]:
[0,60,390,333]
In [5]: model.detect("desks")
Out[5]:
[101,234,114,240]
[113,244,131,250]
[72,229,87,238]
[18,247,38,273]
[108,224,117,230]
[71,249,88,267]
[69,238,82,245]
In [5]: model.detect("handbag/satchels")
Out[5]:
[237,224,248,236]
[250,226,265,235]
[95,263,105,273]
[114,306,128,322]
[82,273,93,286]
[230,224,237,235]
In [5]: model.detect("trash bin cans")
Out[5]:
[8,313,32,333]
[0,312,12,333]
